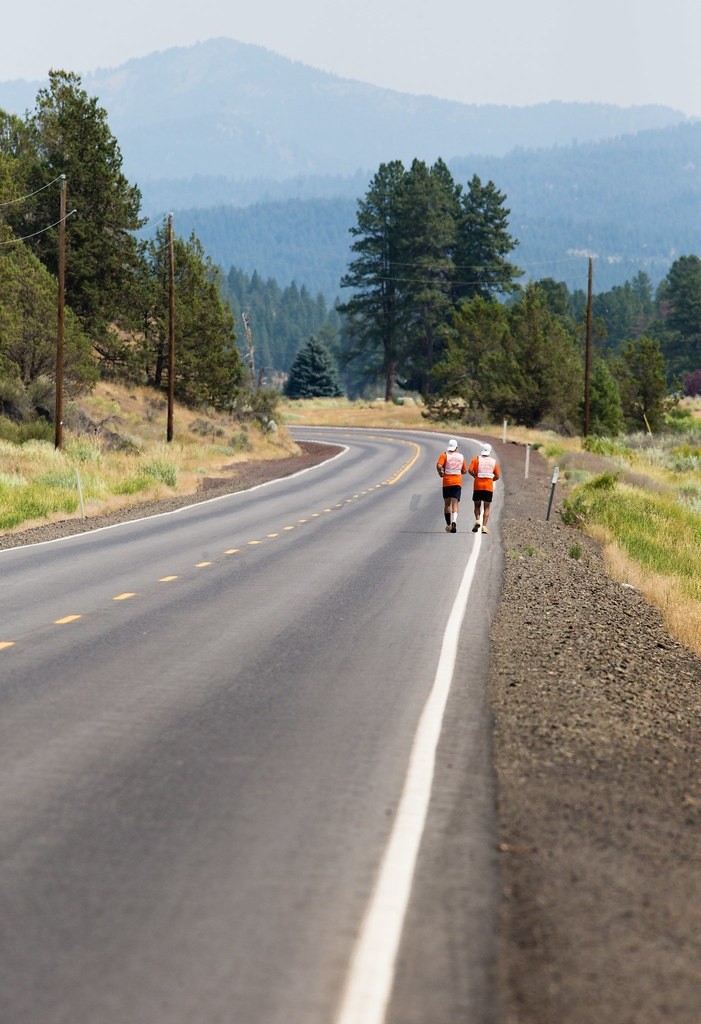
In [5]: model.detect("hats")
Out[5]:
[447,439,458,452]
[480,443,492,455]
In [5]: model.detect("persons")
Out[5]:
[468,443,499,534]
[436,439,467,533]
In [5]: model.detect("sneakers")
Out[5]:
[450,521,458,533]
[446,524,452,532]
[481,526,489,533]
[472,520,481,532]
